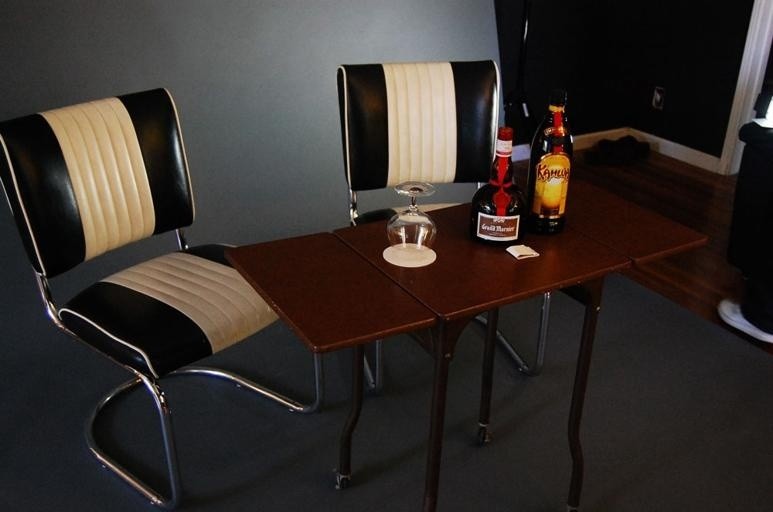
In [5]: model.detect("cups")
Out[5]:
[386,180,437,254]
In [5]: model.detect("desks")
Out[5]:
[223,183,709,510]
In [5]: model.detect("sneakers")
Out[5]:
[717,299,773,343]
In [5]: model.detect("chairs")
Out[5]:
[0,87,325,509]
[337,61,553,396]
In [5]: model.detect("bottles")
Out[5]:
[523,90,574,236]
[470,126,527,247]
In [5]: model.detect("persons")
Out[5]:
[716,149,773,346]
